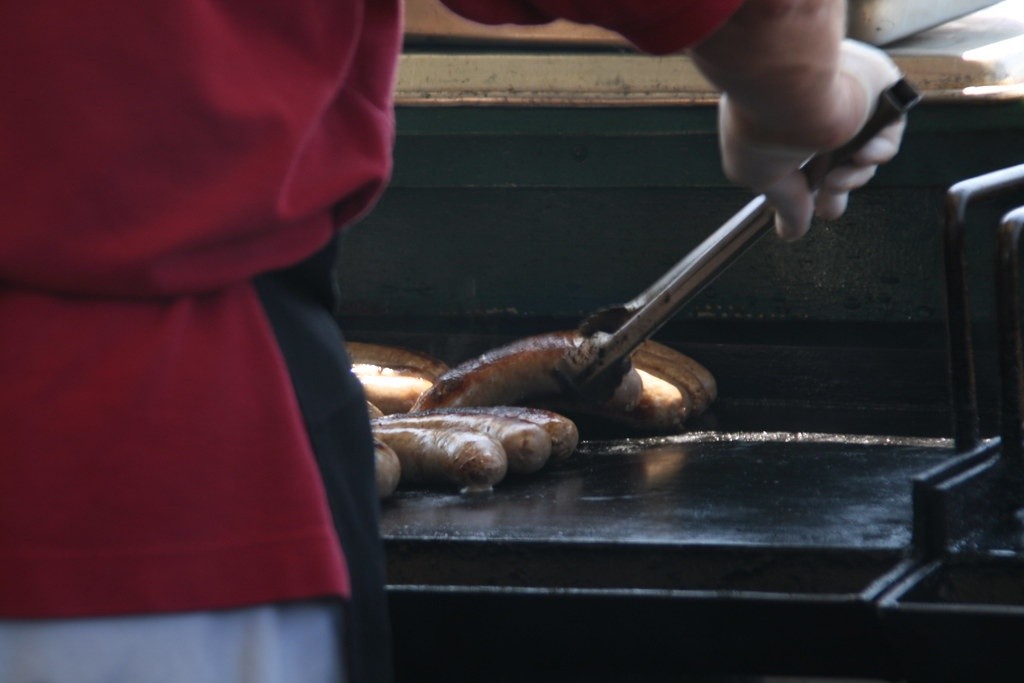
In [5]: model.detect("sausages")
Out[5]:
[344,329,718,498]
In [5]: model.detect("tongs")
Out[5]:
[550,81,922,408]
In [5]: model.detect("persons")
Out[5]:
[0,1,909,683]
